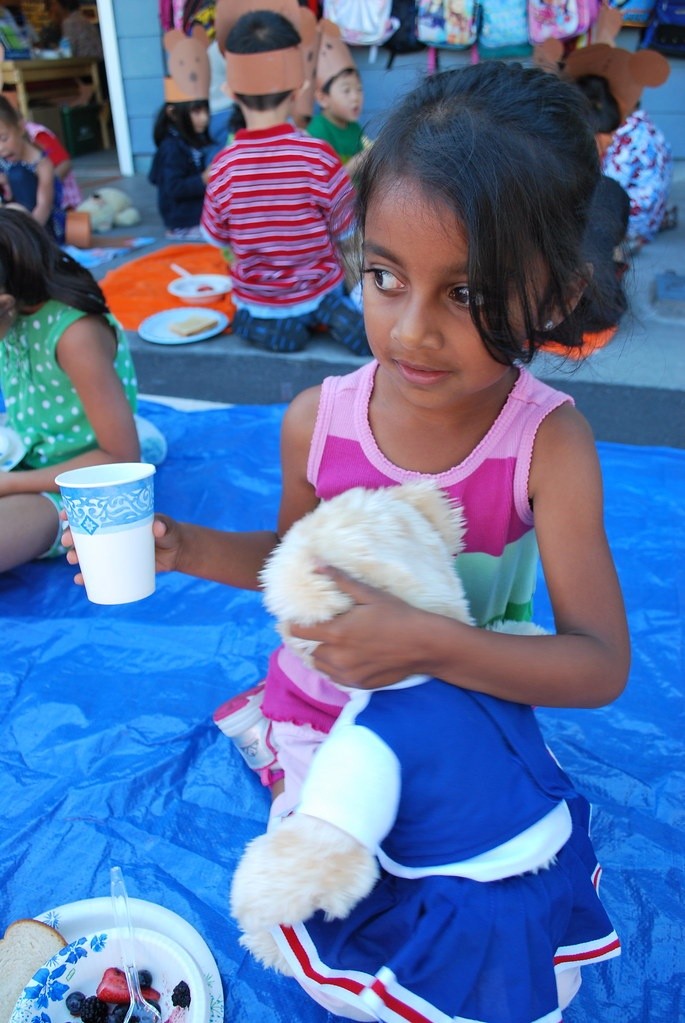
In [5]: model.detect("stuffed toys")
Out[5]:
[227,479,622,1023]
[75,189,142,233]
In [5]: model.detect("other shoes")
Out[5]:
[316,295,374,358]
[231,309,309,353]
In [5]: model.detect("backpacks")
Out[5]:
[321,0,685,52]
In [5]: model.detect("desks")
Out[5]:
[0,57,111,157]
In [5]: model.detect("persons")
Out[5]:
[0,201,145,573]
[1,12,680,357]
[60,64,632,814]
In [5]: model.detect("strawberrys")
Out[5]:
[96,968,162,1003]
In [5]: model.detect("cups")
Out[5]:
[54,462,157,605]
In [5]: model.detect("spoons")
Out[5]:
[108,865,162,1022]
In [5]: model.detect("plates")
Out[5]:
[9,927,210,1023]
[137,306,229,344]
[166,273,234,305]
[0,897,225,1023]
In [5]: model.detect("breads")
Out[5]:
[168,316,217,336]
[0,918,67,1023]
